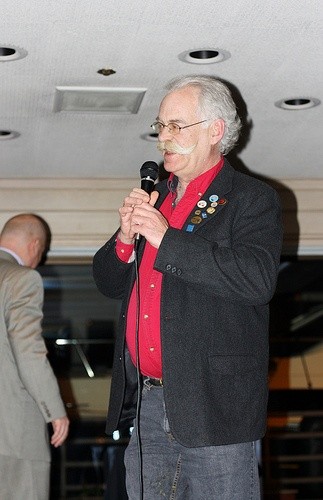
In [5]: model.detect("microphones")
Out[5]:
[134,161,158,251]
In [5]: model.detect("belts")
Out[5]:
[142,376,163,386]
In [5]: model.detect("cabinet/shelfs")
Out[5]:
[58,410,323,500]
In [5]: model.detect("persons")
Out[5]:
[92,75,285,500]
[0,213,69,500]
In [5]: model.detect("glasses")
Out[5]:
[150,120,207,135]
